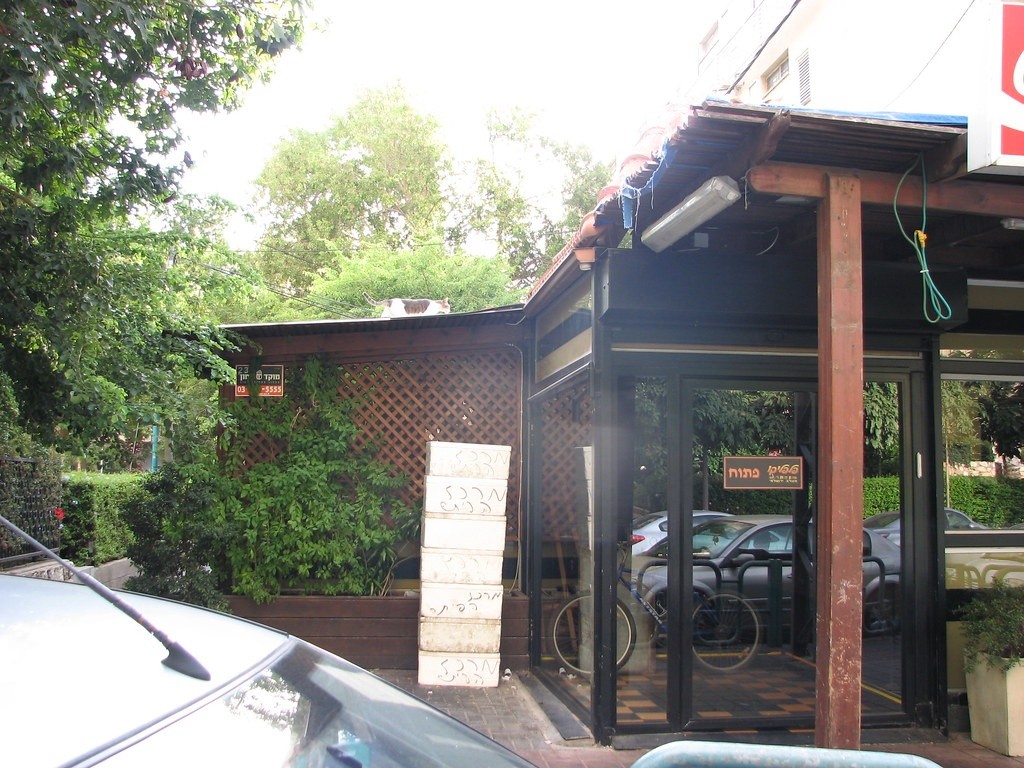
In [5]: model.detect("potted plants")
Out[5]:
[211,356,420,671]
[953,577,1024,757]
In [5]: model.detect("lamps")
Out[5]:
[640,176,739,253]
[999,217,1024,230]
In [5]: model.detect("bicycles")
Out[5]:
[547,534,764,685]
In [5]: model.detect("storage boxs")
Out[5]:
[417,439,511,687]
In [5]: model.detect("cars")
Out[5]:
[862,508,987,548]
[632,509,793,556]
[1,573,542,768]
[632,513,902,642]
